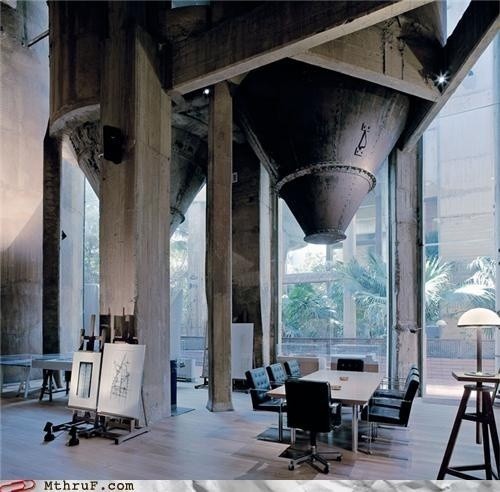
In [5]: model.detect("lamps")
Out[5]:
[457,307,500,376]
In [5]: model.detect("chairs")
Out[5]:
[245,358,421,474]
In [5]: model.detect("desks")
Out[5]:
[0,356,58,399]
[31,354,73,403]
[436,371,500,481]
